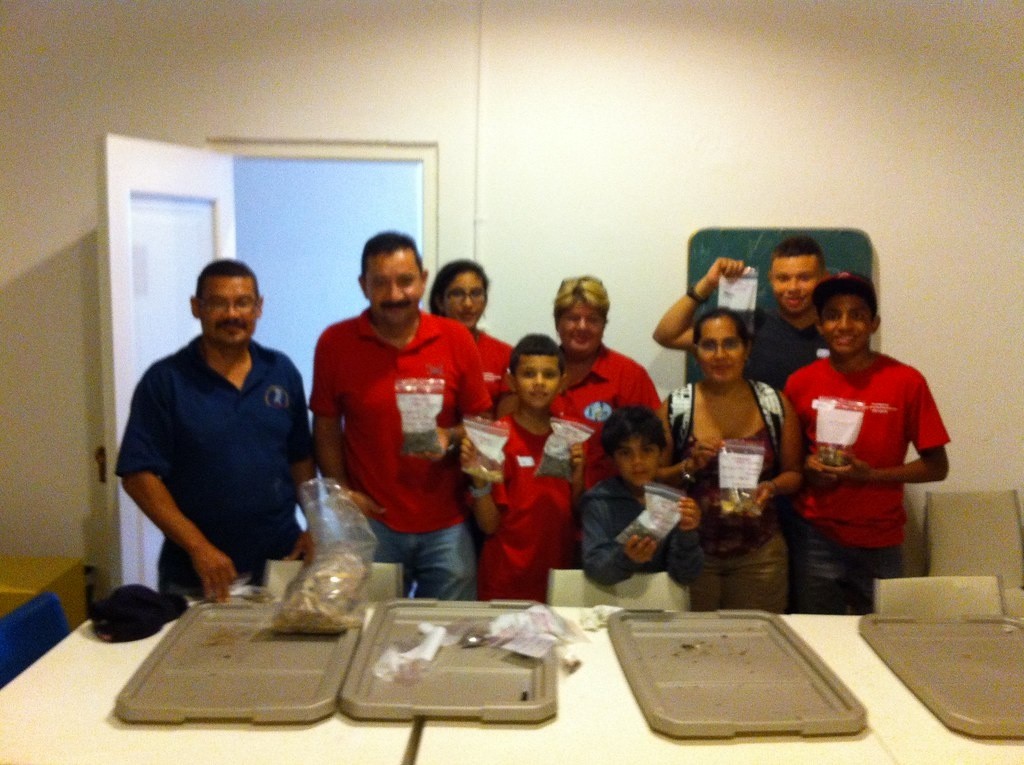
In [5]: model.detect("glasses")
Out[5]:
[444,289,486,301]
[691,340,744,354]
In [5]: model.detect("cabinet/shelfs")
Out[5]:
[0,553,88,632]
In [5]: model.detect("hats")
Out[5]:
[811,271,877,320]
[93,583,189,643]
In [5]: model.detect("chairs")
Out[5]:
[548,567,693,615]
[872,574,1003,618]
[264,560,404,608]
[0,590,75,689]
[923,489,1024,592]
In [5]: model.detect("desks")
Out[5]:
[0,601,1024,765]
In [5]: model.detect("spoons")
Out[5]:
[459,630,489,649]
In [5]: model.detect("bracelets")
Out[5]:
[348,489,353,497]
[683,458,695,482]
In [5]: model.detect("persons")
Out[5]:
[580,405,705,587]
[308,230,494,603]
[461,334,592,605]
[653,235,829,397]
[783,272,952,613]
[430,259,515,400]
[114,260,323,607]
[496,274,662,568]
[656,306,803,609]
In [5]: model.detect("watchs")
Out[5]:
[471,481,492,498]
[686,287,705,302]
